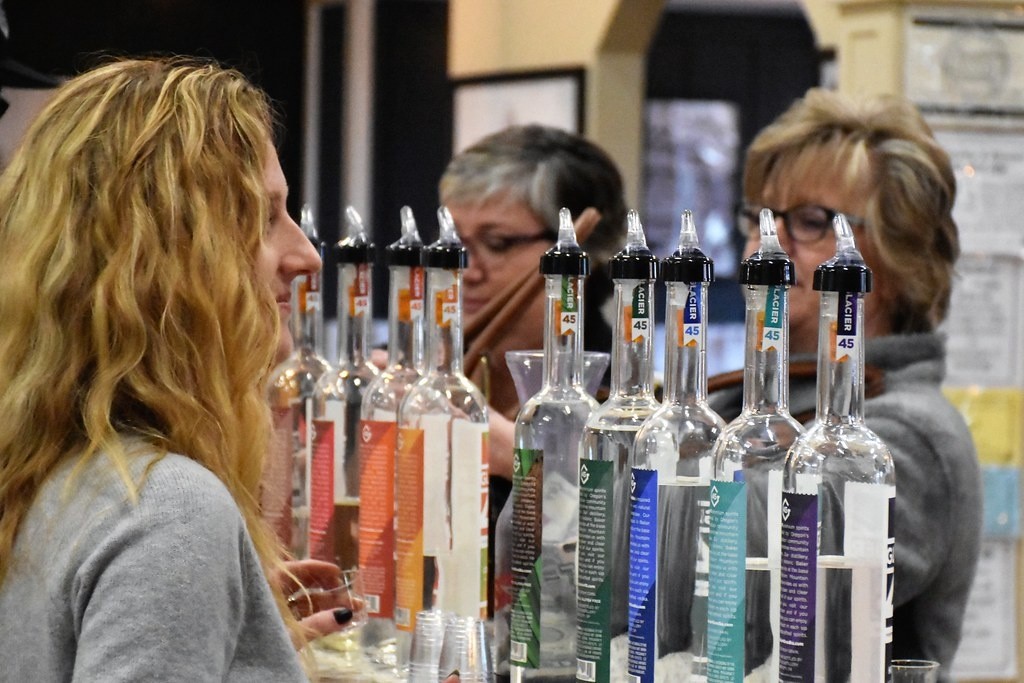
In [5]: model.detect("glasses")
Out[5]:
[733,198,865,244]
[460,228,560,267]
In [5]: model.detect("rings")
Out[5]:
[287,596,303,623]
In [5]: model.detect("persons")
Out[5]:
[357,86,984,683]
[368,123,630,624]
[1,58,369,683]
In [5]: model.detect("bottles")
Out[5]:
[309,207,382,568]
[262,202,330,560]
[394,206,489,677]
[779,213,896,683]
[358,205,425,665]
[507,207,602,683]
[495,349,609,677]
[705,208,806,683]
[574,210,661,683]
[628,208,729,683]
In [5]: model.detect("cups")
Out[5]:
[310,570,369,632]
[408,610,496,683]
[891,660,941,683]
